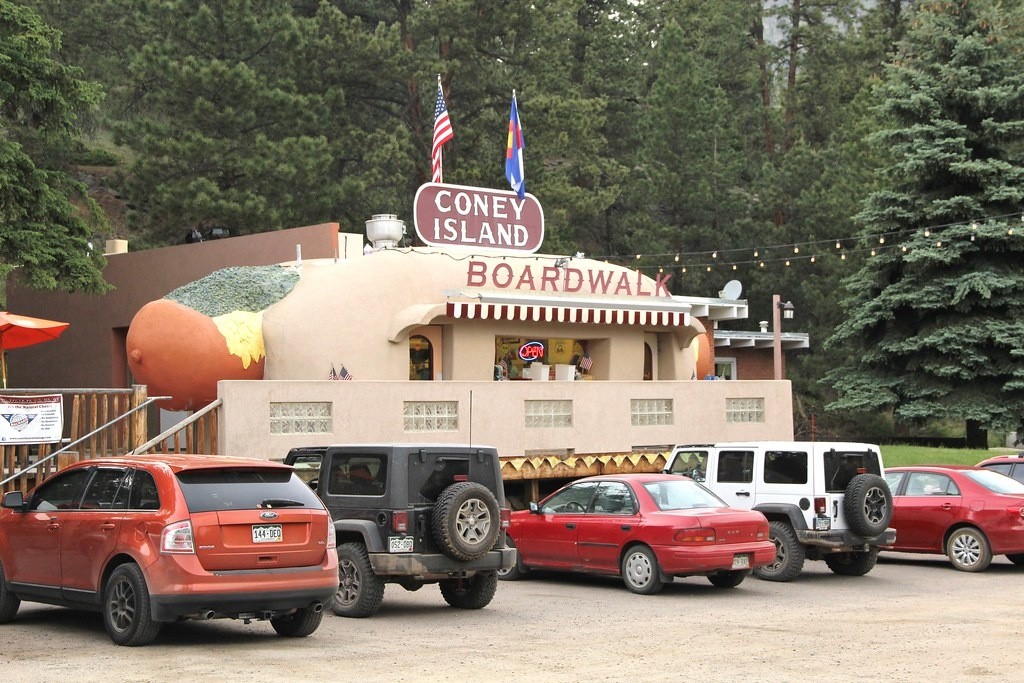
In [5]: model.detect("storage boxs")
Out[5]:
[530,364,552,380]
[555,363,577,381]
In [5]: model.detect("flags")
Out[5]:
[505,88,526,199]
[431,73,454,183]
[329,364,353,381]
[500,356,506,370]
[579,351,593,371]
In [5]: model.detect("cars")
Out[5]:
[498,471,778,596]
[0,453,340,647]
[877,464,1024,573]
[974,452,1024,486]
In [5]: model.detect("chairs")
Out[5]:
[602,489,625,514]
[910,479,924,494]
[343,464,378,495]
[59,477,102,508]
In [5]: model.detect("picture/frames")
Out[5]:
[502,338,520,344]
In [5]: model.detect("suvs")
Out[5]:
[660,440,898,584]
[283,442,519,620]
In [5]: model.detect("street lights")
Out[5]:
[770,293,795,380]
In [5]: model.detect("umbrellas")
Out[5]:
[1,312,70,389]
